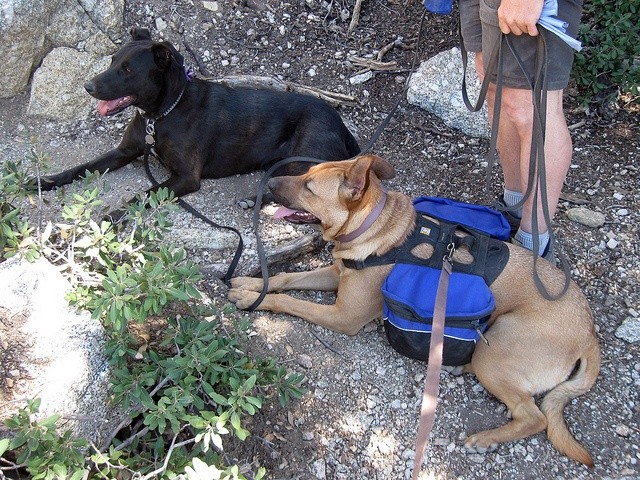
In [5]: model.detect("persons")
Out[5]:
[457,0,586,272]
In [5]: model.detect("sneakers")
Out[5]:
[507,236,556,267]
[490,195,521,233]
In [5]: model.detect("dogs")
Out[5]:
[228,154,602,469]
[24,27,364,231]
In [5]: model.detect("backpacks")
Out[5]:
[341,196,511,367]
